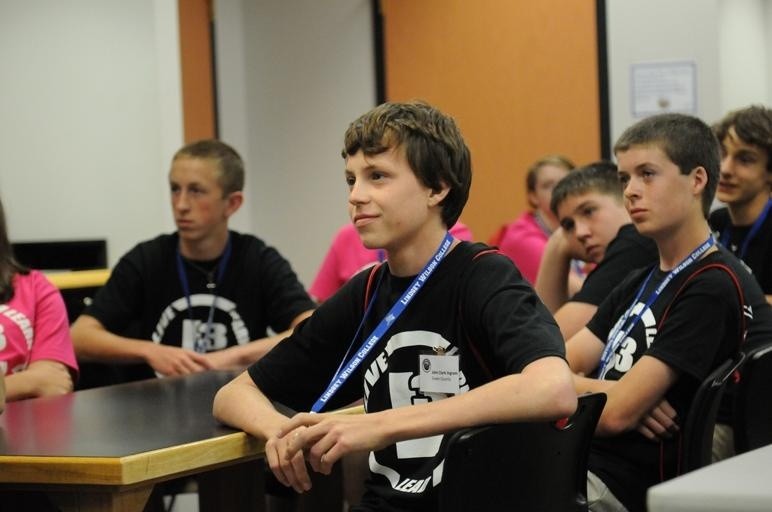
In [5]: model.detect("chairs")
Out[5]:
[74,294,197,512]
[679,346,770,474]
[440,391,609,512]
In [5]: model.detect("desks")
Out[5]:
[0,364,367,512]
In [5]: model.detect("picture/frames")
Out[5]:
[629,60,699,118]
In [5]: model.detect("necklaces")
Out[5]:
[188,262,218,283]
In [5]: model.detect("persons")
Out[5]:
[75,141,318,510]
[0,200,81,398]
